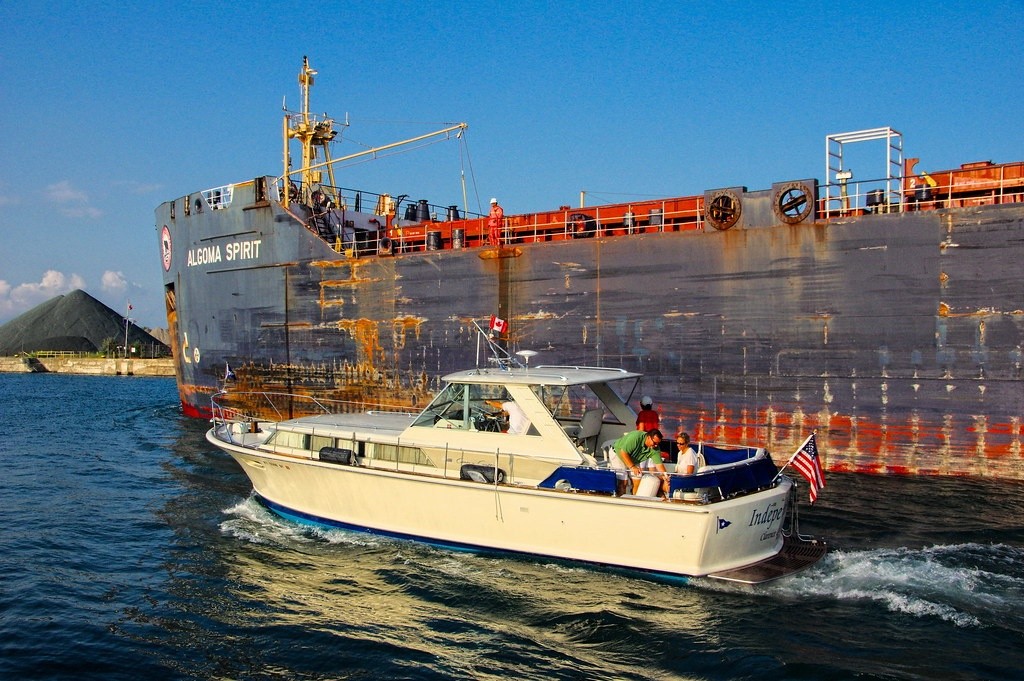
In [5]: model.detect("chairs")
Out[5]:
[561,407,606,457]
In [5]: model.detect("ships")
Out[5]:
[154,55,1024,481]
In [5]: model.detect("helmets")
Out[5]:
[490,198,498,204]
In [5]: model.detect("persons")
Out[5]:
[664,432,698,493]
[484,399,530,435]
[635,396,659,472]
[609,428,669,495]
[487,198,503,245]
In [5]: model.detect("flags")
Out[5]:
[226,364,237,384]
[489,315,507,333]
[789,434,826,505]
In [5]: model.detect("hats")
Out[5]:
[641,395,653,407]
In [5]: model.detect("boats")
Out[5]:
[205,318,828,584]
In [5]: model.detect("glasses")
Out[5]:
[676,441,687,447]
[650,436,660,446]
